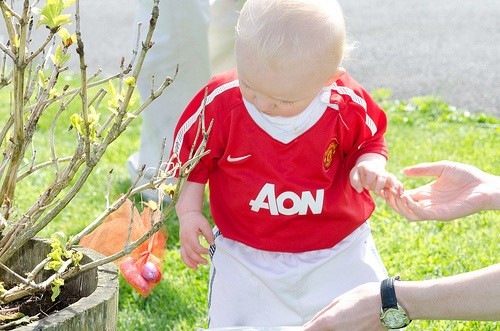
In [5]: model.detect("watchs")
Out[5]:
[379,276,412,329]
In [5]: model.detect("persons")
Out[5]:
[164,0,405,331]
[300,160,500,331]
[129,0,245,205]
